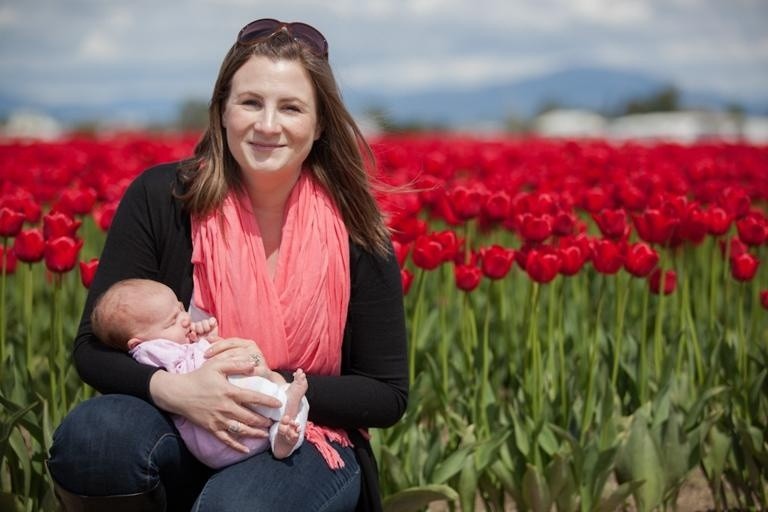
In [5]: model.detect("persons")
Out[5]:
[45,20,409,512]
[90,278,309,470]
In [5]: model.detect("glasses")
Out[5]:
[234,17,328,61]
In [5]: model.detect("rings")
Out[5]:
[229,422,240,434]
[249,351,262,364]
